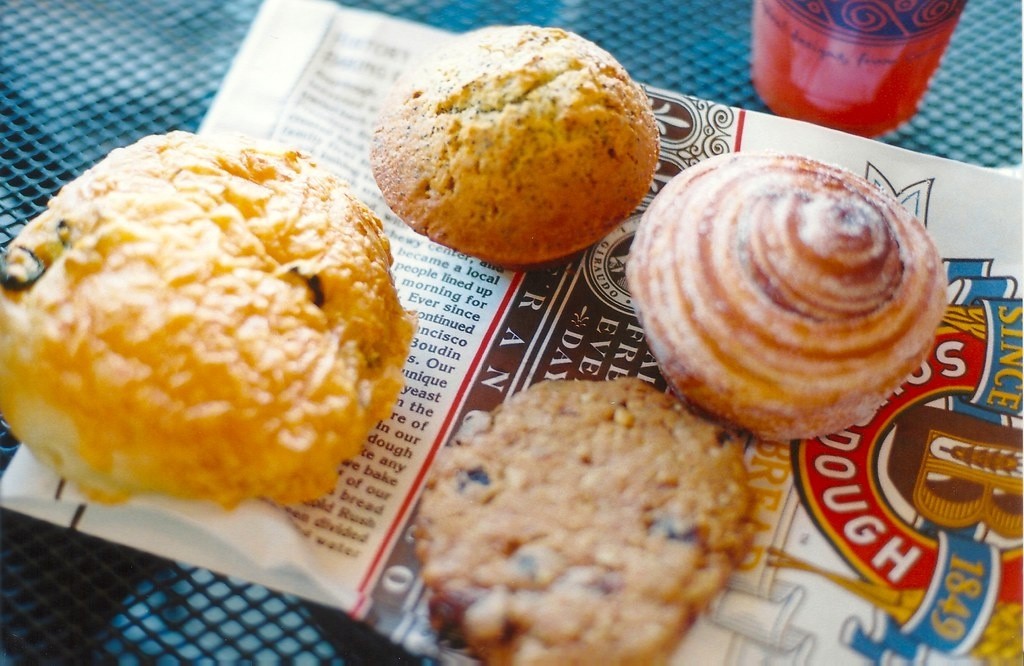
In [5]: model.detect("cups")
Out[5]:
[752,0,967,138]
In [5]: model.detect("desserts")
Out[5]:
[629,151,949,440]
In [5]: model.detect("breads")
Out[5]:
[369,24,658,265]
[0,131,416,504]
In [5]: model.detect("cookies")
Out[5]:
[413,376,755,666]
